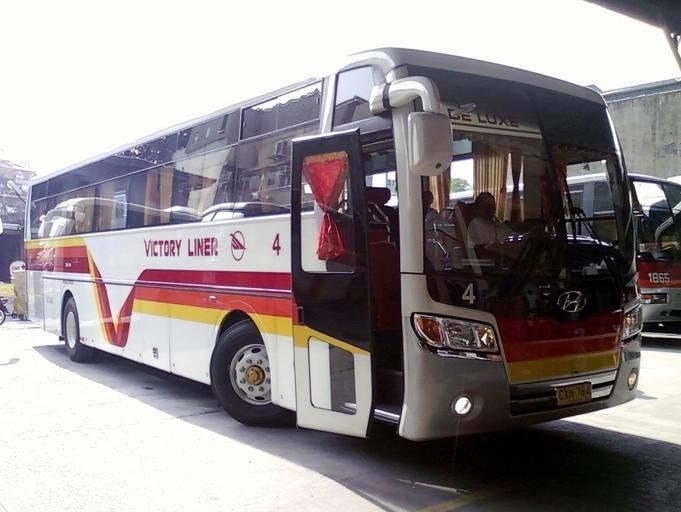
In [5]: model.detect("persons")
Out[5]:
[426,190,438,223]
[467,191,521,251]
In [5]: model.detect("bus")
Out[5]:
[23,46,643,442]
[387,171,680,340]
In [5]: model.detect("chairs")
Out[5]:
[454,201,498,272]
[365,187,395,218]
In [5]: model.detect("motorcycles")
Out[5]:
[0,295,9,325]
